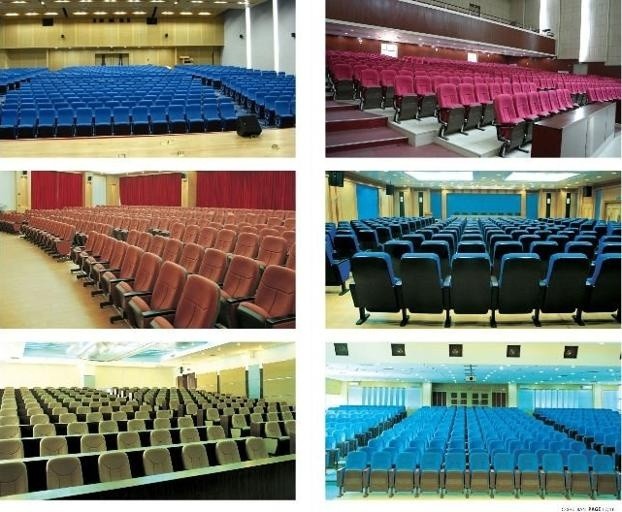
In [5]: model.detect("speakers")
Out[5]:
[43,18,53,26]
[146,16,157,24]
[236,115,262,136]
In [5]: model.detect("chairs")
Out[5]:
[326,48,621,158]
[0,386,296,497]
[1,207,295,329]
[325,404,621,501]
[325,211,621,329]
[0,64,296,139]
